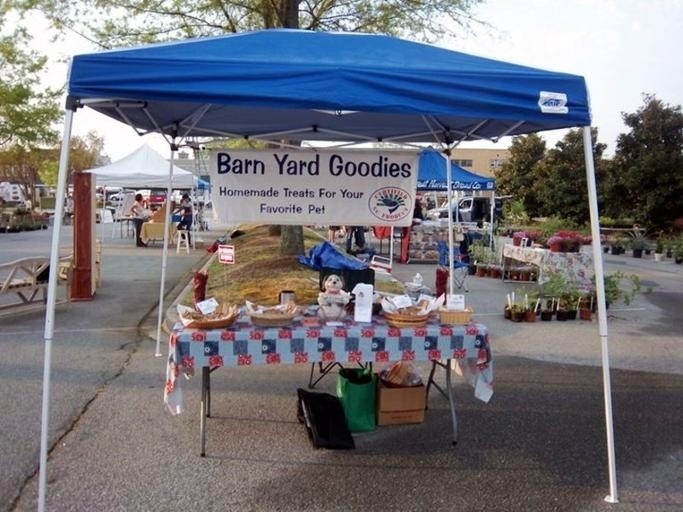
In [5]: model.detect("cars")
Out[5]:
[0,180,190,232]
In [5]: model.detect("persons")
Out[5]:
[343,225,354,255]
[427,197,435,210]
[130,193,148,248]
[413,199,425,221]
[355,227,369,248]
[173,194,192,246]
[193,203,209,231]
[326,225,340,245]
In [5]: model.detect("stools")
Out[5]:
[172,228,193,255]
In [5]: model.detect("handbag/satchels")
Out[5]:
[297,388,355,450]
[336,367,375,431]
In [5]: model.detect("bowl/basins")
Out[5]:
[246,311,296,326]
[348,302,381,316]
[383,310,427,328]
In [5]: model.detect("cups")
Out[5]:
[277,290,296,304]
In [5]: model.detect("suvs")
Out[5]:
[425,196,514,222]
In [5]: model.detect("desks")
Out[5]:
[111,214,149,241]
[158,308,498,461]
[137,215,178,247]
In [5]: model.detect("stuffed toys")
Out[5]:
[315,273,350,325]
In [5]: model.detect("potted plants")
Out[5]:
[470,223,641,321]
[626,224,682,263]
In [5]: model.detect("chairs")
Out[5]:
[434,239,471,294]
[305,266,375,390]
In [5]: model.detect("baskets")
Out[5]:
[438,305,474,325]
[176,303,240,329]
[384,307,433,328]
[245,301,296,326]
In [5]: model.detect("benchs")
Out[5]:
[0,238,101,318]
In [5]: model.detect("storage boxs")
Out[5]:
[372,375,428,426]
